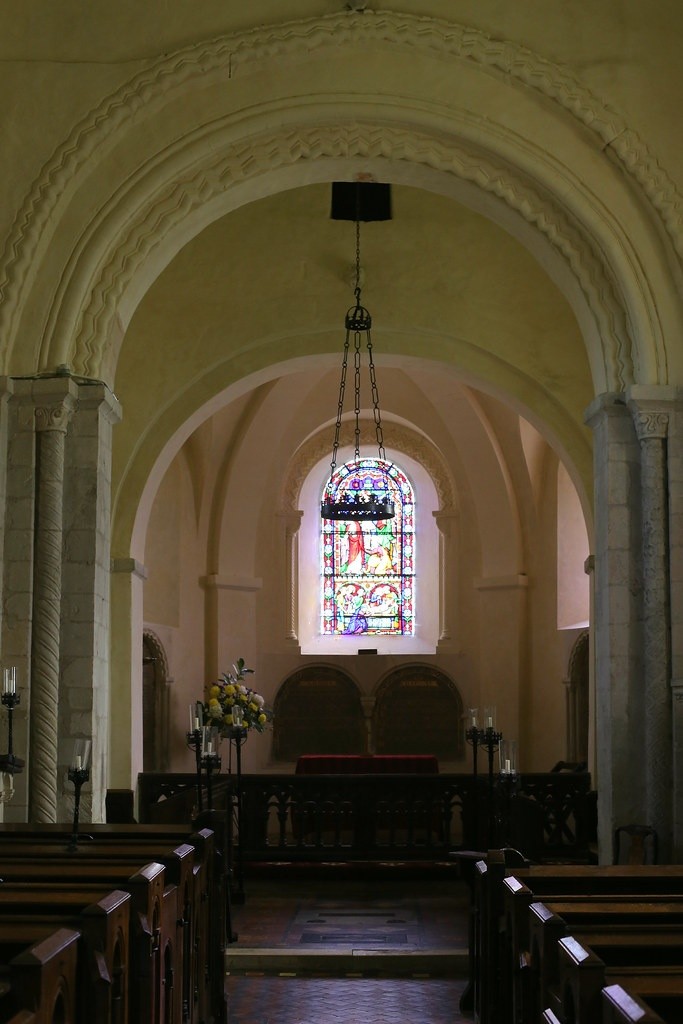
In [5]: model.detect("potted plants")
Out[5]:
[75,756,81,770]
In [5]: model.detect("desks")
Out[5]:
[295,754,439,773]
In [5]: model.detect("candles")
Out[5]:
[9,679,13,693]
[195,718,198,730]
[208,742,212,753]
[472,717,475,726]
[488,716,492,728]
[189,705,192,736]
[506,760,511,774]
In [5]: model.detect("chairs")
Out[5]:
[613,824,659,864]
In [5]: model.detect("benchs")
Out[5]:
[0,823,228,1024]
[468,847,683,1024]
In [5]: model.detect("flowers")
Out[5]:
[195,656,275,741]
